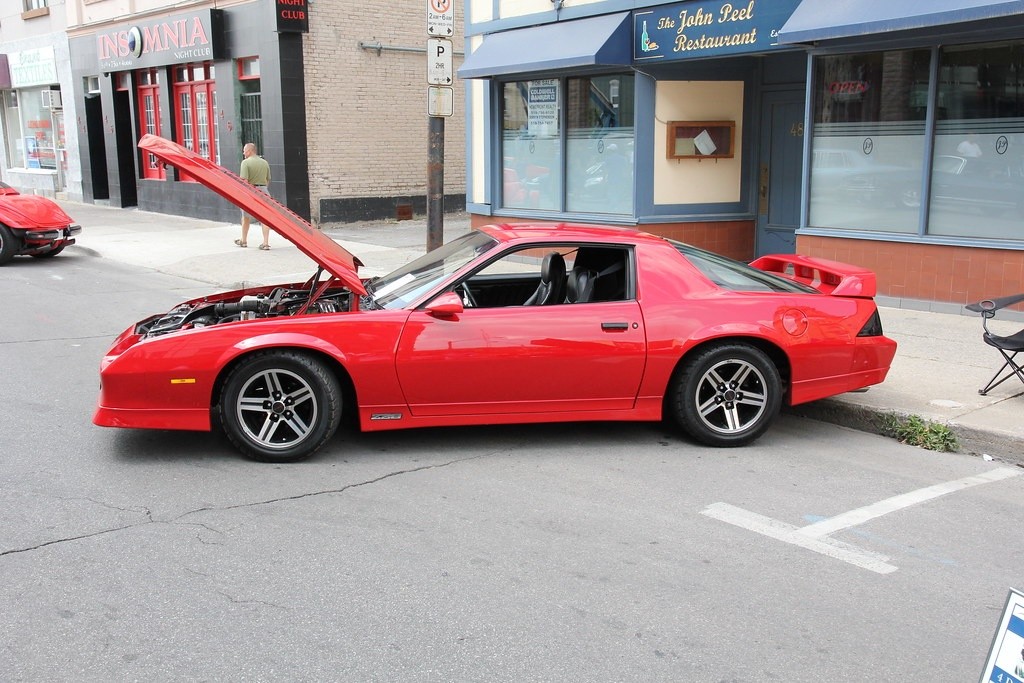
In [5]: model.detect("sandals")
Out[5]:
[259,244,272,251]
[236,239,248,247]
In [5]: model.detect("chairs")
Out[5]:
[965,293,1024,395]
[522,252,568,306]
[562,265,591,304]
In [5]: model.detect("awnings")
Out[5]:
[457,11,632,79]
[779,0,1024,47]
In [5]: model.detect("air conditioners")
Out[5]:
[41,90,62,108]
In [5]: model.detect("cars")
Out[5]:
[901,155,1024,213]
[810,146,912,205]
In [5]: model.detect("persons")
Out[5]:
[234,143,271,250]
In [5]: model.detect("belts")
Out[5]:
[254,184,266,186]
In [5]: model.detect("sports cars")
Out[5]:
[90,134,898,463]
[0,181,81,266]
